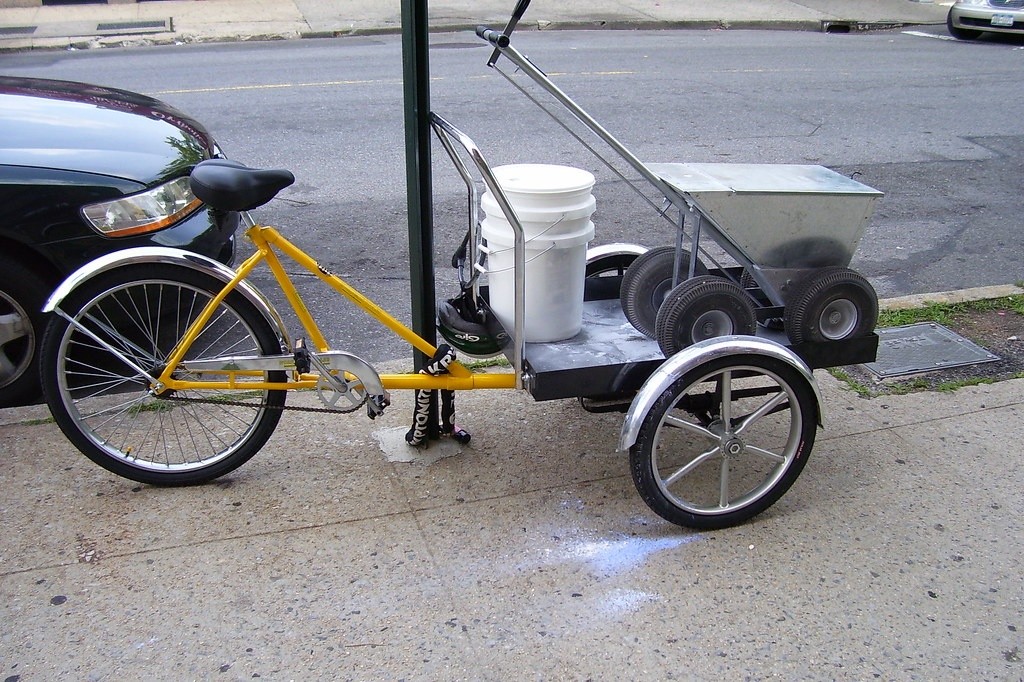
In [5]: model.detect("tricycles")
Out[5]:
[34,155,880,532]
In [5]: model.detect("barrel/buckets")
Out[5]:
[473,164,597,344]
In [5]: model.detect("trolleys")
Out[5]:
[474,0,880,358]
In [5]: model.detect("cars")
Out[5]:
[0,75,241,412]
[947,1,1024,41]
